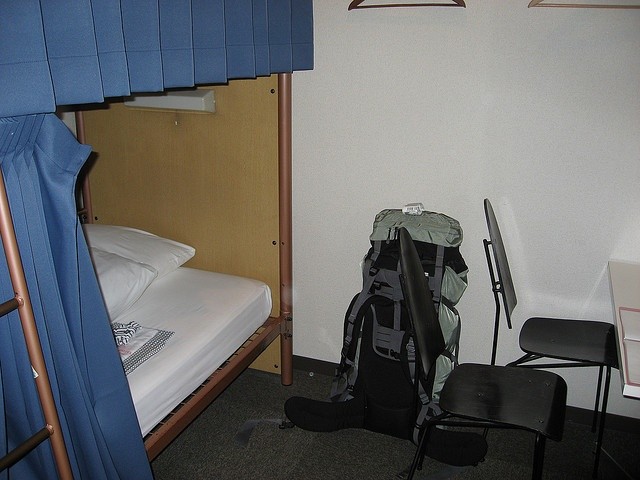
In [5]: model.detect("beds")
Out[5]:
[0,1,314,480]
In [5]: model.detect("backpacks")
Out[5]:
[330,208,468,446]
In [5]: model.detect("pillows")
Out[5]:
[81,223,196,277]
[88,246,159,321]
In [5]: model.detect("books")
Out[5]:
[619,307,640,388]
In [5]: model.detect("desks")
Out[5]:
[607,259,640,401]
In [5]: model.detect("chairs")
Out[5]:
[483,198,619,479]
[396,226,567,480]
